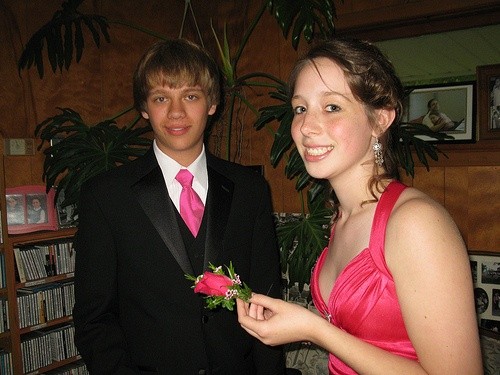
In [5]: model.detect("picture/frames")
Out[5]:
[405,80,477,144]
[5,186,57,234]
[476,64,500,140]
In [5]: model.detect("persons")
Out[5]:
[74,37,287,375]
[237,36,484,375]
[493,79,500,115]
[7,196,21,224]
[423,99,454,131]
[28,198,45,223]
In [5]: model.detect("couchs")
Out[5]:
[284,300,500,375]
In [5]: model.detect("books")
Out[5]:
[0,325,89,375]
[0,239,76,288]
[0,211,3,243]
[0,282,75,333]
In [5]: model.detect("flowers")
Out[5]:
[184,260,252,311]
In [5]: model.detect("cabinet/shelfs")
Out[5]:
[0,227,89,375]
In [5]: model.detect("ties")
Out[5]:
[175,169,206,238]
